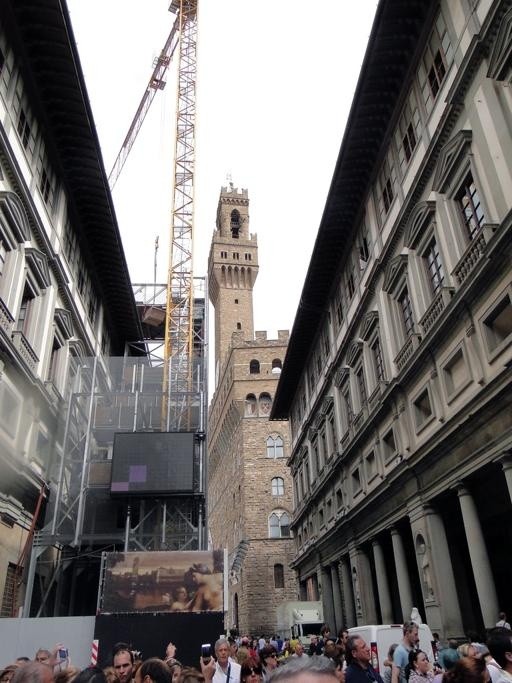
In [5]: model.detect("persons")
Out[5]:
[191,563,224,612]
[170,585,197,612]
[352,573,362,614]
[0,611,512,682]
[420,543,435,602]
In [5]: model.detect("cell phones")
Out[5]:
[59,648,68,658]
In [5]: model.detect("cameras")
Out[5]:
[135,651,142,658]
[201,644,212,663]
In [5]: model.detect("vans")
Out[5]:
[346,624,439,683]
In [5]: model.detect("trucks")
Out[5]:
[276,601,325,654]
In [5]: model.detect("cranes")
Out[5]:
[106,0,199,551]
